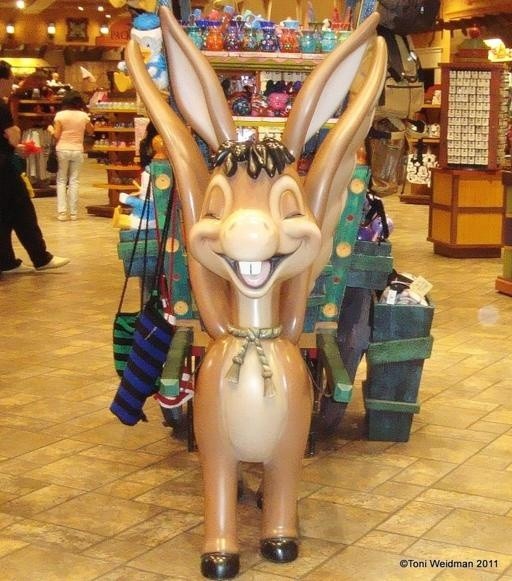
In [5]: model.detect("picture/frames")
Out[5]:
[66,17,89,42]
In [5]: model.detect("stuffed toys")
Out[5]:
[111,11,170,93]
[112,166,154,230]
[224,79,297,117]
[355,195,396,244]
[122,0,392,581]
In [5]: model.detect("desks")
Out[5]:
[427,167,505,258]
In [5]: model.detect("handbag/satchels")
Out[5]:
[47,146,58,172]
[110,297,174,425]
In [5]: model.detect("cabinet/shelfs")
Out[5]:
[408,103,443,144]
[4,51,350,216]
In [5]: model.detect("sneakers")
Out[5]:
[36,256,70,271]
[58,214,77,221]
[2,263,34,273]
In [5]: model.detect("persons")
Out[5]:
[49,90,94,222]
[0,60,69,275]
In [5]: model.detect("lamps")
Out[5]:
[48,21,56,38]
[483,34,508,49]
[7,21,15,35]
[101,21,109,34]
